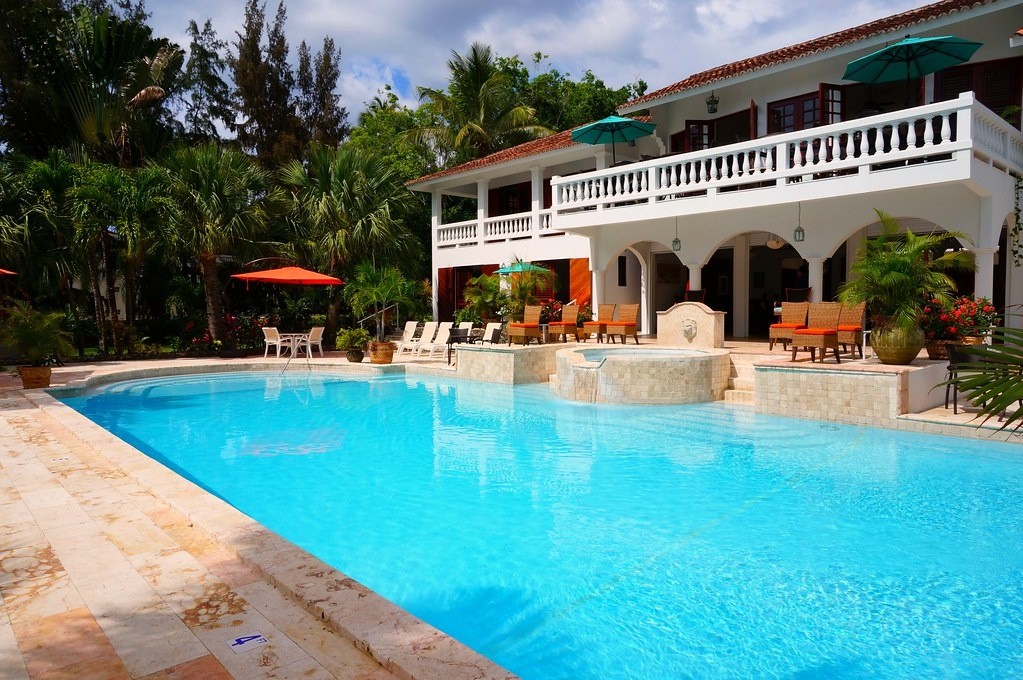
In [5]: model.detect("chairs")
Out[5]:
[302,327,325,359]
[945,344,986,414]
[390,320,502,364]
[769,301,866,364]
[262,327,291,358]
[508,304,639,346]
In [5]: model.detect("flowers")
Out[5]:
[186,313,268,349]
[540,301,562,325]
[920,297,1001,340]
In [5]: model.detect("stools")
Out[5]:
[774,287,812,345]
[539,324,549,344]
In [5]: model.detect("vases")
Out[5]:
[926,337,986,360]
[538,329,560,343]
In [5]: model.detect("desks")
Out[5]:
[281,333,313,357]
[451,335,481,366]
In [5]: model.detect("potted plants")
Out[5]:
[578,306,593,338]
[0,296,79,389]
[833,208,979,365]
[336,328,370,362]
[345,262,415,363]
